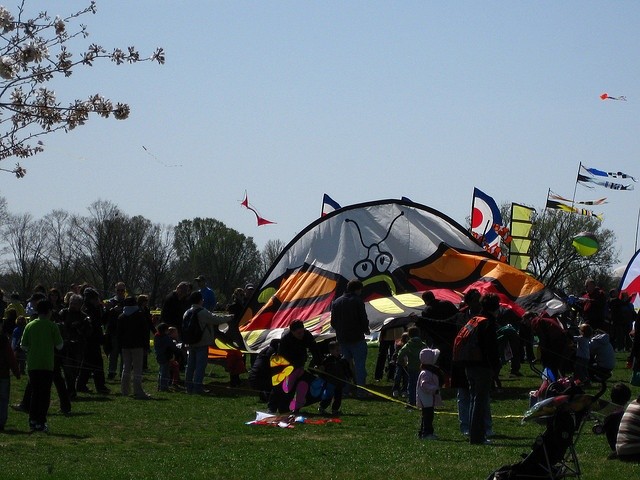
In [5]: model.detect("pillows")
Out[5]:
[520,393,620,423]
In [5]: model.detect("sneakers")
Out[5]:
[393,390,398,396]
[422,433,438,440]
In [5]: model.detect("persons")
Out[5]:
[396,327,429,408]
[317,340,353,418]
[605,383,631,452]
[390,332,410,397]
[503,279,640,381]
[1,281,156,433]
[410,289,481,436]
[454,293,502,446]
[268,318,321,417]
[615,395,640,462]
[421,290,458,342]
[330,280,375,399]
[247,338,281,401]
[416,348,442,440]
[154,274,253,394]
[374,326,405,382]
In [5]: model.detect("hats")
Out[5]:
[26,292,47,302]
[419,347,441,365]
[245,284,255,290]
[194,275,207,283]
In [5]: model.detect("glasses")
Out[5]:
[115,288,125,292]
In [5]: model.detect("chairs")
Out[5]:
[486,369,606,480]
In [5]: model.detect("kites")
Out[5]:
[208,187,571,372]
[600,93,627,102]
[245,410,343,428]
[240,189,277,227]
[270,353,524,418]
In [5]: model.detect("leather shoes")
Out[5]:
[7,403,28,414]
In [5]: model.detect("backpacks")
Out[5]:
[452,315,489,371]
[180,307,208,345]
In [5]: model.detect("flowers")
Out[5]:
[539,372,580,393]
